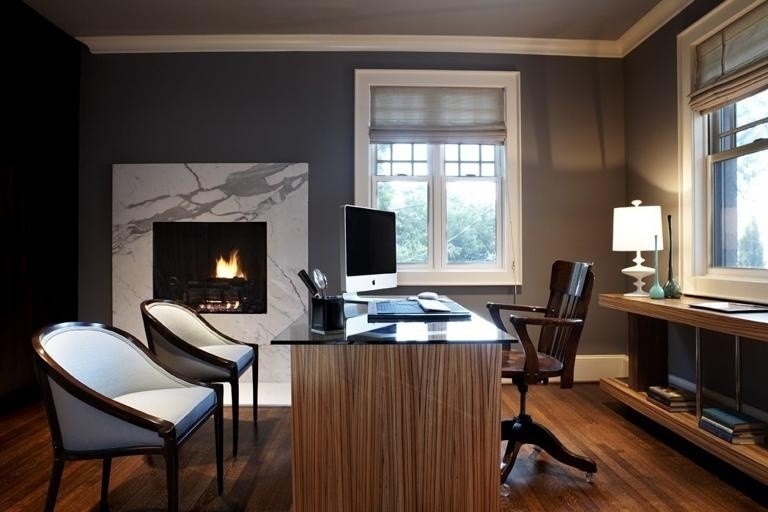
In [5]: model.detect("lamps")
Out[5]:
[613,198,665,299]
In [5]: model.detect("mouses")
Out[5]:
[417,291,439,299]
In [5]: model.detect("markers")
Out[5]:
[297,269,321,300]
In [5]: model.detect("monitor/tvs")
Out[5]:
[339,203,398,303]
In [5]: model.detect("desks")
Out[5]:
[273,292,520,512]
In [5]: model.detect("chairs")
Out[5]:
[141,298,259,461]
[485,259,598,497]
[31,320,224,512]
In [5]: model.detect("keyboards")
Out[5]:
[417,298,450,312]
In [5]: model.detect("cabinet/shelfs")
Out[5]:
[599,290,768,483]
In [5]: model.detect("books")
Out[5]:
[698,407,768,445]
[646,383,702,413]
